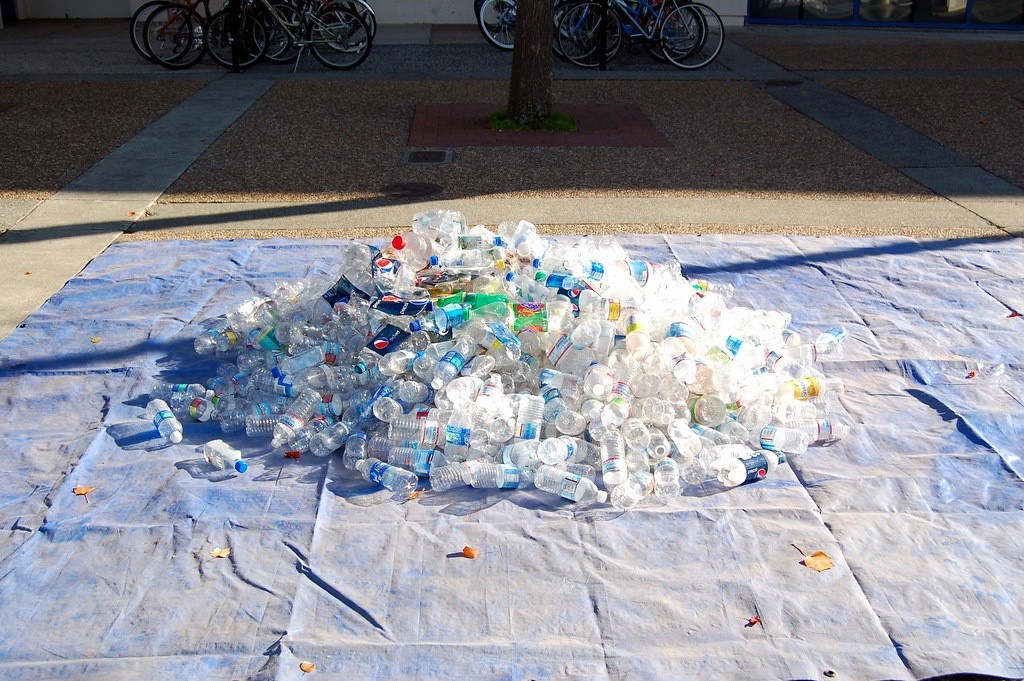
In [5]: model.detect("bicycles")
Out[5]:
[125,0,728,78]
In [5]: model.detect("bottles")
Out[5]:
[144,211,849,508]
